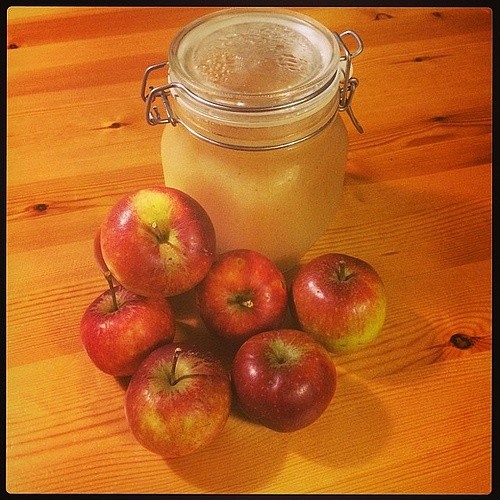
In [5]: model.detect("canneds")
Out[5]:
[141,7,363,273]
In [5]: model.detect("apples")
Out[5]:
[194,249,289,341]
[100,185,215,298]
[289,253,387,354]
[231,330,336,433]
[79,283,176,376]
[124,342,230,457]
[94,226,119,286]
